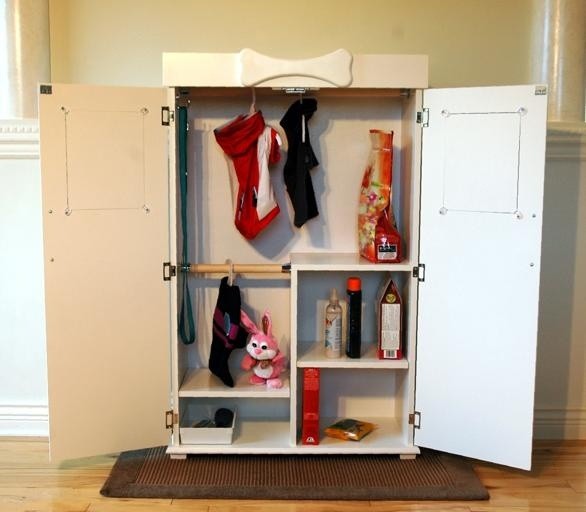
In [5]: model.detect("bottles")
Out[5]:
[325,276,362,359]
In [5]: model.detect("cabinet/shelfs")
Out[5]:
[39,47,547,472]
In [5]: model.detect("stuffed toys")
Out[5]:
[238,308,285,389]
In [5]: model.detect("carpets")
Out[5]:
[98,446,488,500]
